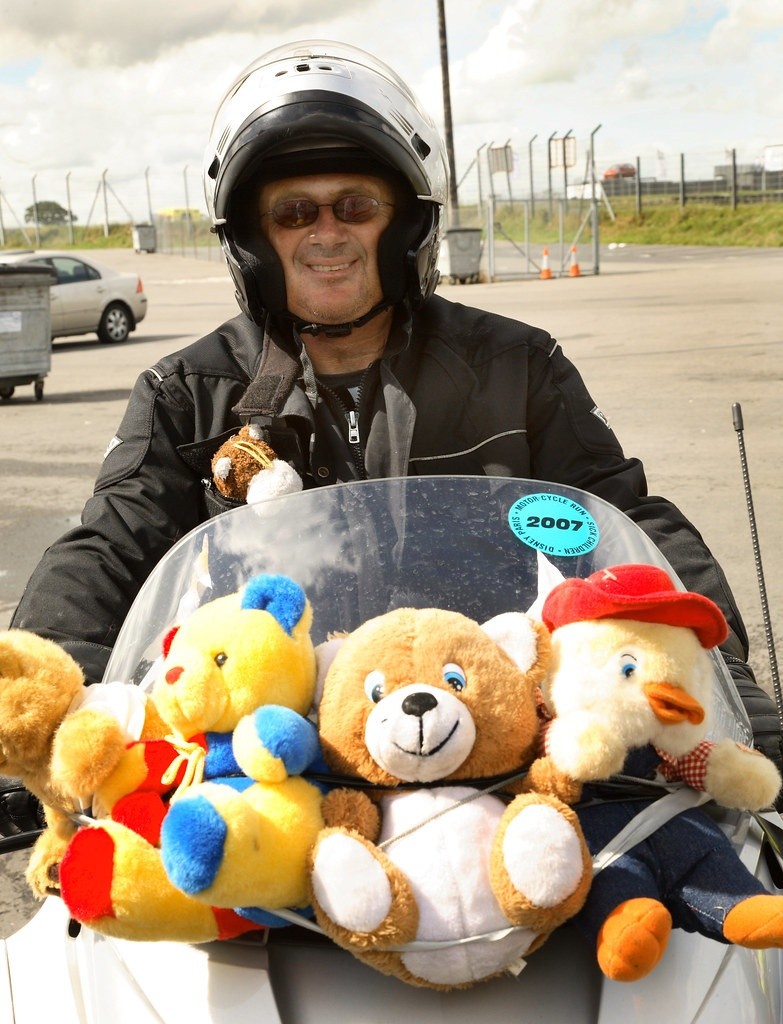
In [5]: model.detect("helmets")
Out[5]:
[200,41,445,333]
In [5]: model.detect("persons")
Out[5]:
[0,31,783,820]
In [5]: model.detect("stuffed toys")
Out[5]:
[1,628,179,904]
[309,604,591,992]
[487,566,783,984]
[212,425,302,515]
[60,576,323,946]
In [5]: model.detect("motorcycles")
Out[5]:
[0,475,782,1024]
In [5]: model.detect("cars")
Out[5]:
[3,253,147,343]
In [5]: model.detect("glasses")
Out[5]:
[250,197,397,227]
